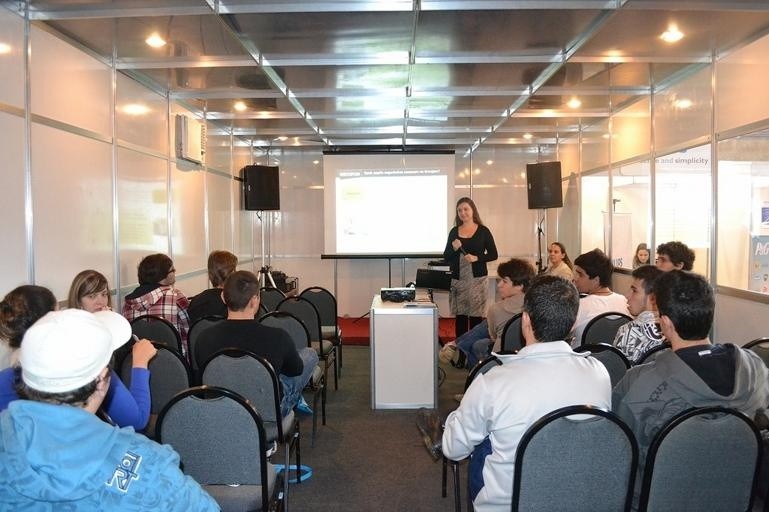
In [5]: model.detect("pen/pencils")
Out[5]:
[133,334,140,342]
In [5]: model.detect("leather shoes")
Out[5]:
[414,407,450,462]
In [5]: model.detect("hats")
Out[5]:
[18,307,133,393]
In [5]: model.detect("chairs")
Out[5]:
[440,307,767,512]
[106,282,344,511]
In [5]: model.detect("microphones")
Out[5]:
[454,239,467,256]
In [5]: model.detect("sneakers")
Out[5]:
[296,398,313,416]
[438,343,455,364]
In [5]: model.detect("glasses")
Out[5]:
[167,267,175,274]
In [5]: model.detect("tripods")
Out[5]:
[257,217,280,292]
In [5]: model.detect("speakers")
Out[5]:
[526,161,562,209]
[242,165,279,210]
